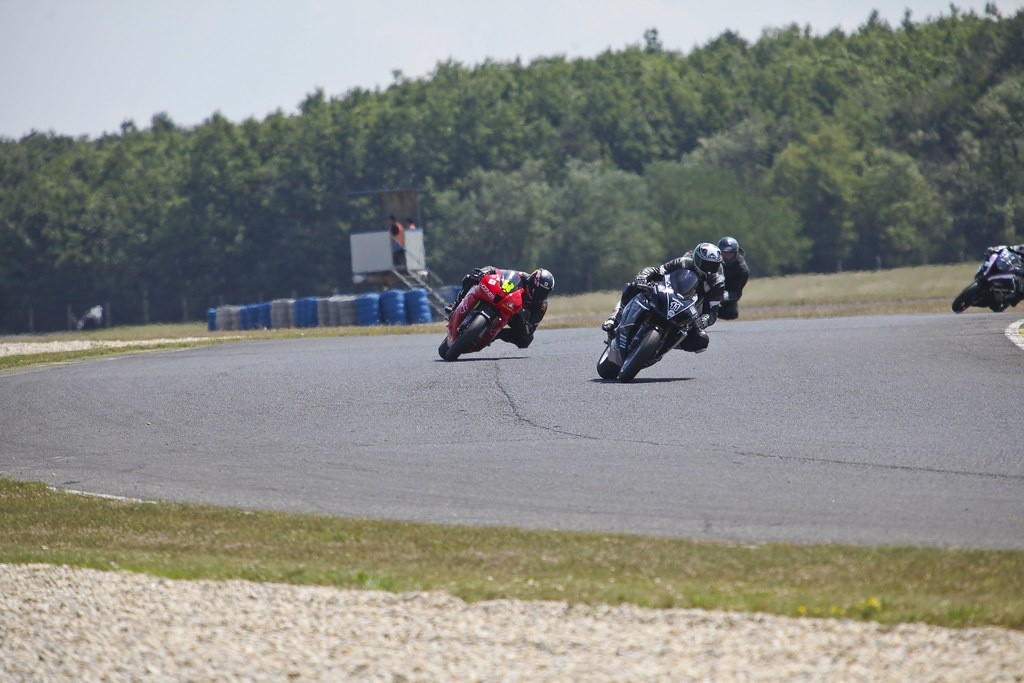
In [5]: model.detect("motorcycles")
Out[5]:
[597,272,703,383]
[949,247,1024,314]
[436,264,534,363]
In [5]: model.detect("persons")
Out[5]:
[984,245,1024,307]
[445,266,554,348]
[390,213,416,266]
[602,237,750,354]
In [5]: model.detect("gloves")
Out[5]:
[469,271,481,284]
[636,275,646,289]
[692,317,703,330]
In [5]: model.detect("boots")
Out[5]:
[445,290,466,315]
[602,300,624,332]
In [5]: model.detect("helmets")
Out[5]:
[717,237,739,266]
[527,269,555,301]
[694,243,722,277]
